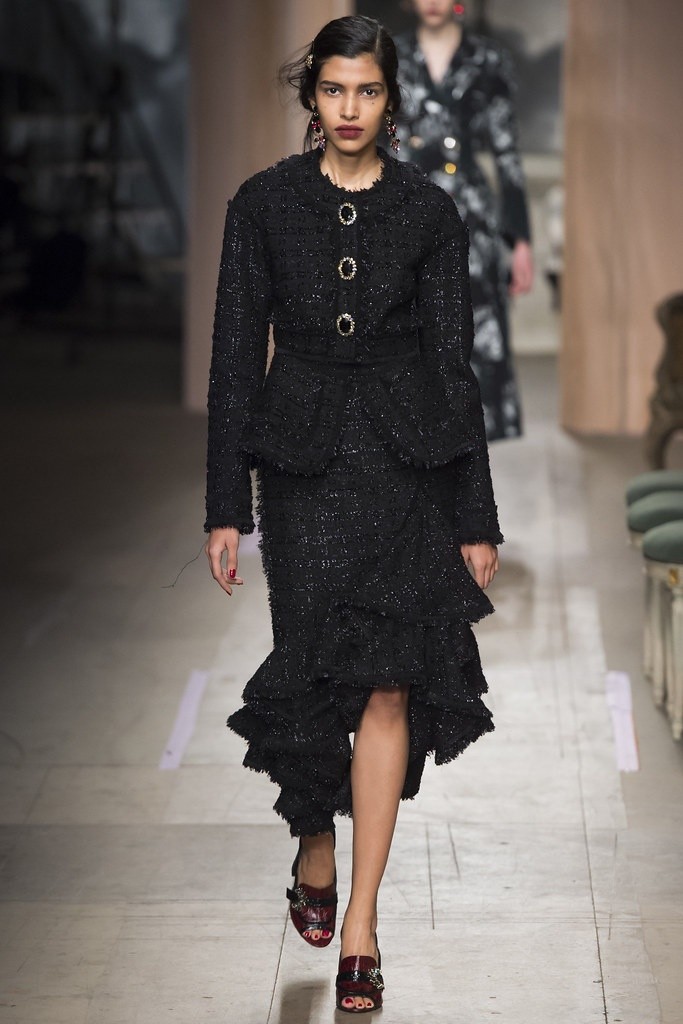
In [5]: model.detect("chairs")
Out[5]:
[624,291,683,468]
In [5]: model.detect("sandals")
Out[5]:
[285,842,338,948]
[335,925,384,1012]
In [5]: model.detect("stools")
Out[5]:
[642,521,683,739]
[627,490,683,706]
[625,469,683,674]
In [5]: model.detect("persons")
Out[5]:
[386,0,542,440]
[203,16,504,1013]
